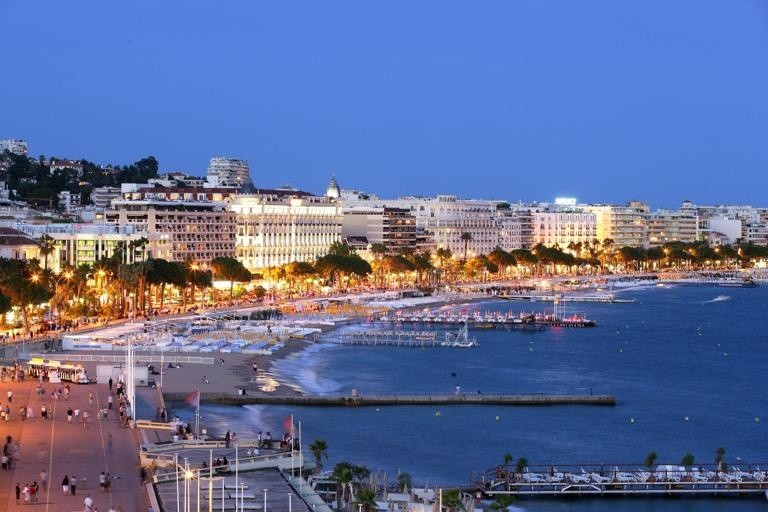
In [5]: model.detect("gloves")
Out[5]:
[524,471,768,482]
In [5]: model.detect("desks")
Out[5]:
[56,273,70,333]
[186,473,192,511]
[94,272,105,287]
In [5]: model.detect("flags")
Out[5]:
[285,417,291,432]
[186,392,198,408]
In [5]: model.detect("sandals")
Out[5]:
[30,358,90,384]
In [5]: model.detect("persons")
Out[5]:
[301,276,410,313]
[1,367,93,424]
[108,435,113,451]
[0,434,19,470]
[16,471,112,511]
[455,383,461,396]
[101,377,132,428]
[475,486,482,507]
[2,322,79,342]
[148,358,258,396]
[143,305,198,321]
[156,407,166,422]
[171,415,298,468]
[140,461,158,490]
